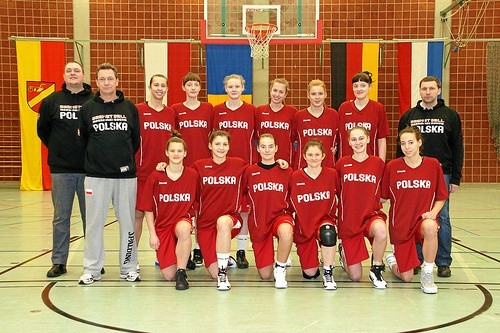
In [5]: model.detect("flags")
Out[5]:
[15,40,68,193]
[205,44,254,106]
[396,42,443,119]
[143,43,190,106]
[329,43,379,110]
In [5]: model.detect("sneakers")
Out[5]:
[368,265,388,289]
[321,264,337,290]
[273,261,288,289]
[385,253,398,271]
[217,267,231,291]
[120,268,141,282]
[193,249,205,267]
[338,244,347,270]
[79,268,102,284]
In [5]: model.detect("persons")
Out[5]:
[76,63,141,285]
[211,74,258,267]
[169,71,214,266]
[381,127,449,294]
[256,77,303,165]
[144,132,202,291]
[156,130,289,292]
[333,127,388,289]
[394,76,464,277]
[283,138,343,290]
[130,74,175,272]
[337,71,391,164]
[37,62,106,278]
[241,133,296,289]
[291,79,340,168]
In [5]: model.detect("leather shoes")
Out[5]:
[236,250,249,269]
[174,269,190,290]
[186,253,197,270]
[47,264,67,277]
[412,258,422,275]
[437,265,451,277]
